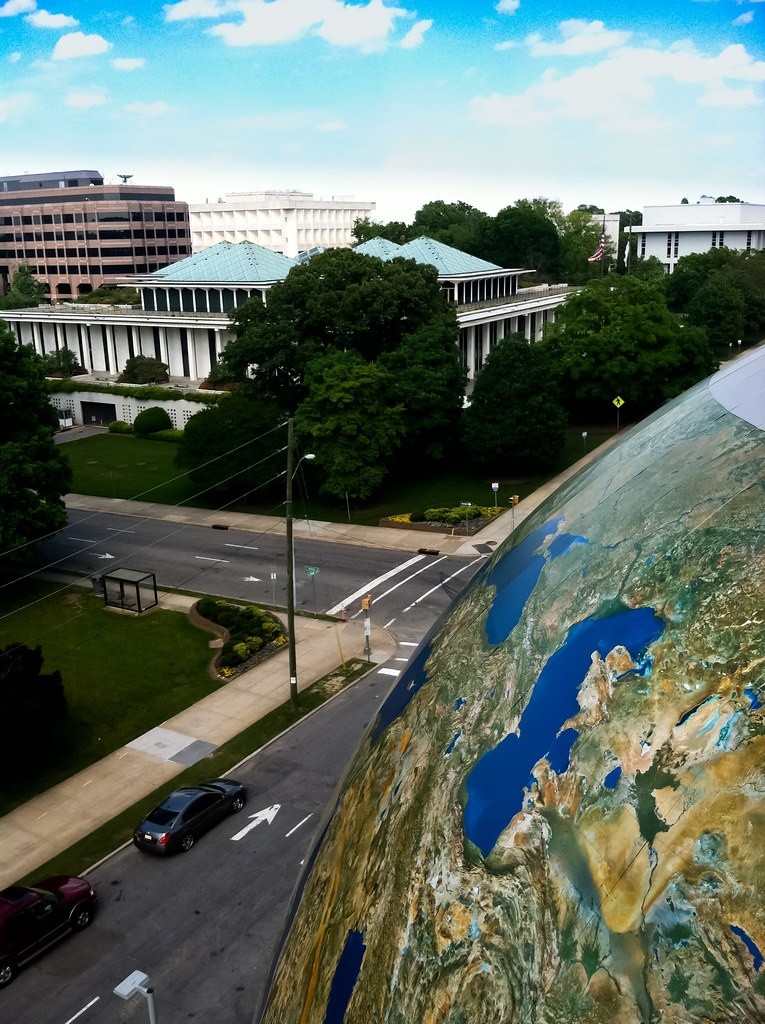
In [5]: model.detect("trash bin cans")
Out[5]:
[90,574,104,593]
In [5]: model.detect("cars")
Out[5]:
[133,778,248,859]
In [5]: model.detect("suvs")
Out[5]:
[0,875,97,989]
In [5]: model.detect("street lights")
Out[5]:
[491,483,499,507]
[291,454,315,607]
[738,339,741,352]
[582,431,587,454]
